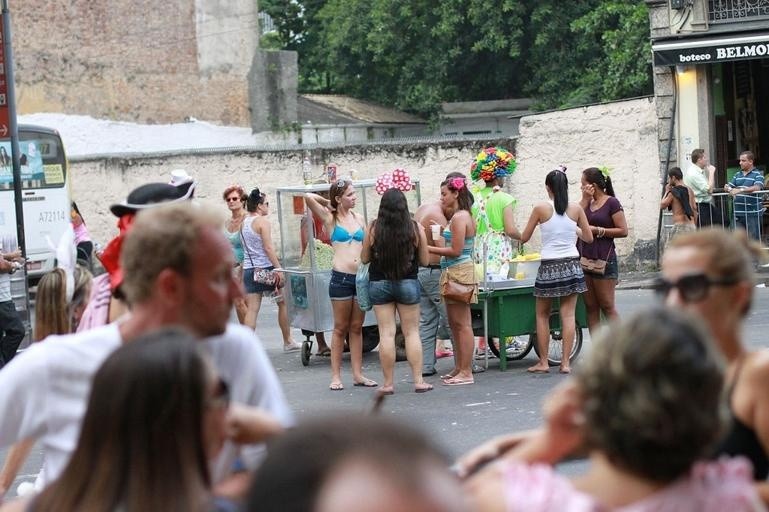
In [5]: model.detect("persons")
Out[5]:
[575,167,628,348]
[724,151,764,273]
[246,404,478,512]
[457,311,769,511]
[685,148,730,229]
[651,226,769,512]
[299,146,521,395]
[1,172,296,511]
[660,166,698,253]
[520,169,593,375]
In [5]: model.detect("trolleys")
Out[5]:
[434,268,609,372]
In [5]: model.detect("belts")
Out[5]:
[427,264,441,269]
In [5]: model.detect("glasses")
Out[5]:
[336,176,353,192]
[226,196,240,202]
[653,272,736,306]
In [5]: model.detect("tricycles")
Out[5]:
[270,261,412,368]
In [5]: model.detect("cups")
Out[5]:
[432,225,440,240]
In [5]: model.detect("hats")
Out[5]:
[470,147,516,182]
[111,169,194,218]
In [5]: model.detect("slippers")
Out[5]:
[283,340,572,395]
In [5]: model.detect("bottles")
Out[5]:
[302,157,312,189]
[499,259,510,281]
[326,162,336,184]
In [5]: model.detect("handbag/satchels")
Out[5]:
[253,267,276,288]
[444,279,474,305]
[580,256,606,276]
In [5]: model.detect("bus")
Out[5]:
[0,124,77,318]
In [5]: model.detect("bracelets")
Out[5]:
[596,226,606,239]
[740,187,744,193]
